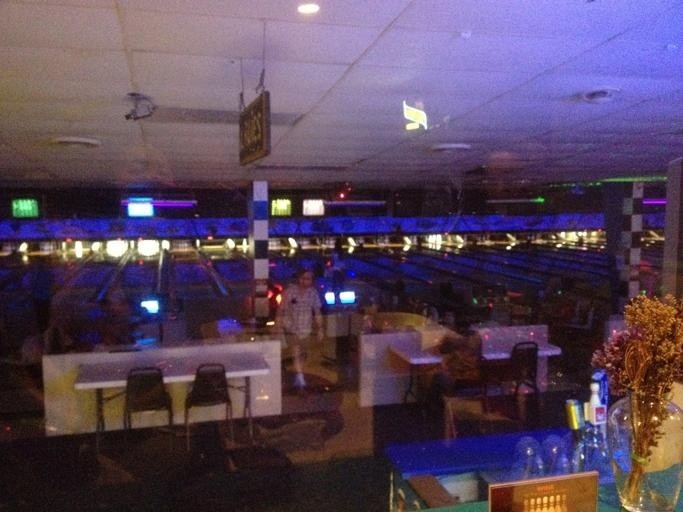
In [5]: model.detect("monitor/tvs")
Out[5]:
[268,198,294,221]
[11,198,39,219]
[140,300,160,315]
[124,197,154,219]
[300,198,326,217]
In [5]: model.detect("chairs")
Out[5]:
[425,340,543,419]
[123,362,236,454]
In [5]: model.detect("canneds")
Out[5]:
[565,397,584,432]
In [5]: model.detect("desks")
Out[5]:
[73,351,270,462]
[388,339,561,414]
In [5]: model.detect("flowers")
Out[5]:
[590,291,683,503]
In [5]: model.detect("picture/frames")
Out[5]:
[238,92,269,166]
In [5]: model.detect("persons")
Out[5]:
[272,266,325,394]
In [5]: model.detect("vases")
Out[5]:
[604,394,682,511]
